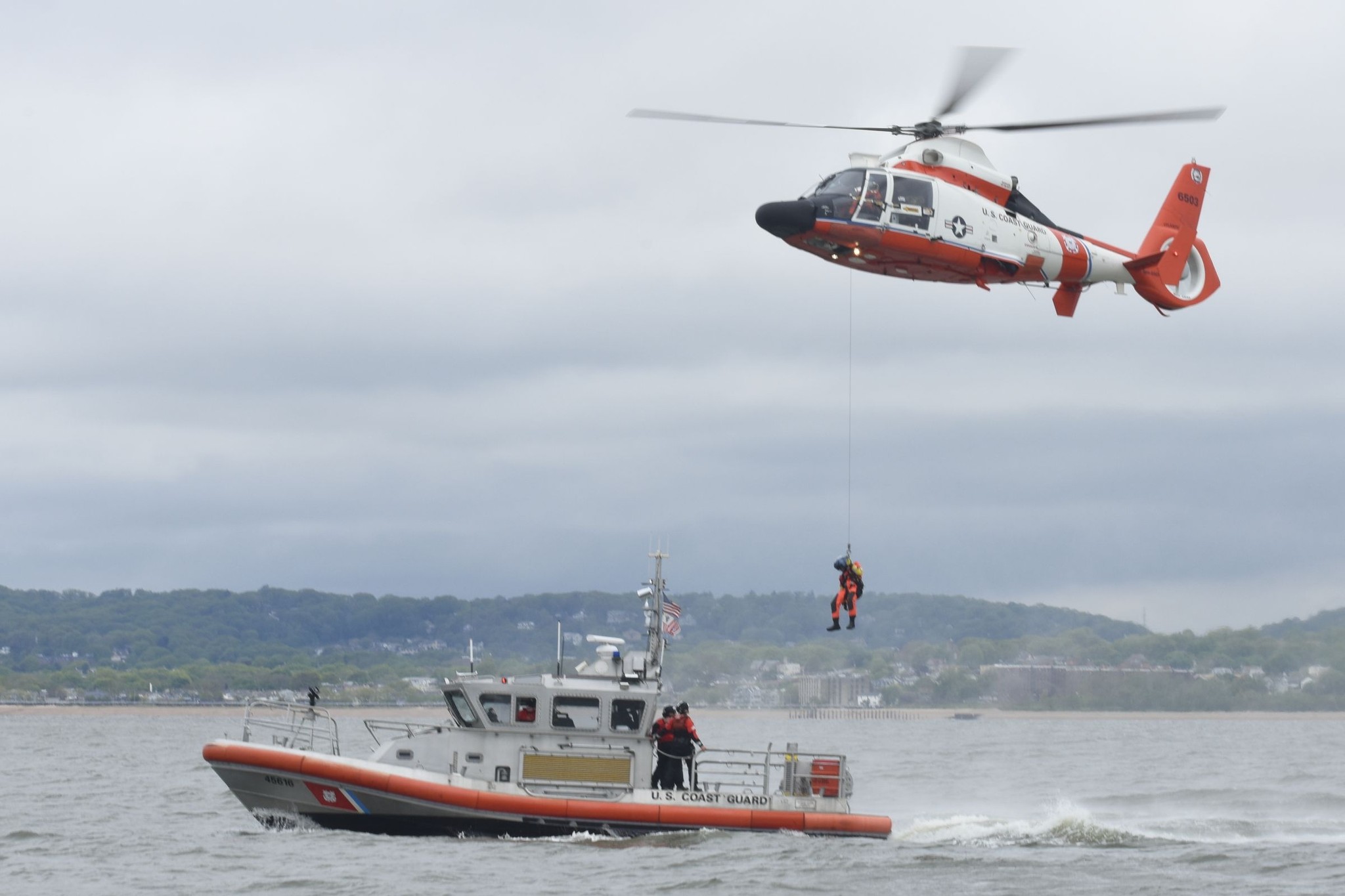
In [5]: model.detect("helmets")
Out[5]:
[676,701,689,714]
[834,557,846,571]
[662,705,676,717]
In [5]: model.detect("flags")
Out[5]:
[662,591,683,638]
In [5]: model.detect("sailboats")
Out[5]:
[626,43,1229,322]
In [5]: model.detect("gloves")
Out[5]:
[846,558,852,567]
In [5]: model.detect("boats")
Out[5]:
[197,549,897,845]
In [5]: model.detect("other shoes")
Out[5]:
[661,784,669,790]
[676,786,689,791]
[846,622,855,629]
[826,623,841,631]
[651,780,658,789]
[692,786,702,791]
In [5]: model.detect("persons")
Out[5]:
[826,555,864,632]
[650,700,707,791]
